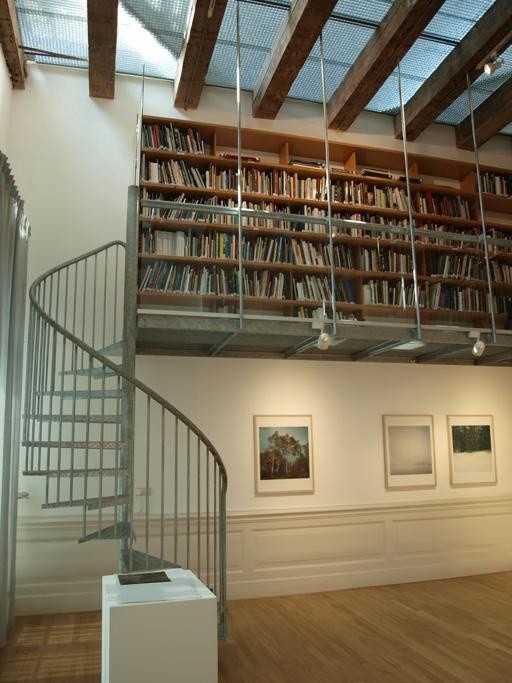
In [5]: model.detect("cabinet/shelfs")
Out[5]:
[132,111,512,318]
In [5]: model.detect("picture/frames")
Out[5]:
[253,415,315,497]
[383,413,498,491]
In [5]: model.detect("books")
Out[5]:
[220,153,261,162]
[289,159,325,170]
[331,167,353,173]
[240,165,332,320]
[474,172,512,198]
[415,191,491,312]
[395,176,424,184]
[331,177,415,306]
[139,123,240,314]
[359,168,393,179]
[486,229,512,314]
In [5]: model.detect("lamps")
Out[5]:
[484,52,506,76]
